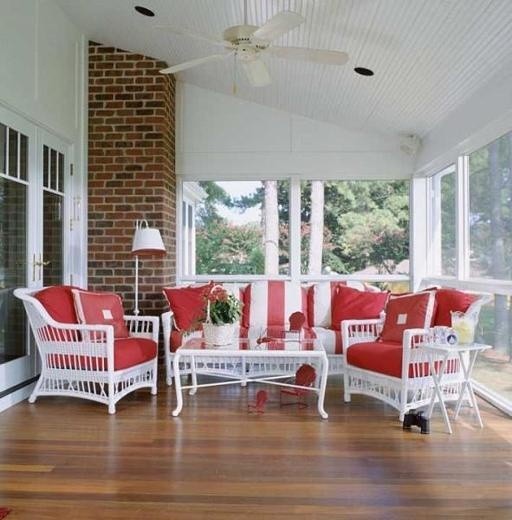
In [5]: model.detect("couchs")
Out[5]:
[162,281,388,384]
[14,285,160,414]
[340,285,496,422]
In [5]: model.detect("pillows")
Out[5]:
[73,290,130,340]
[165,280,220,332]
[431,286,475,333]
[245,281,309,332]
[304,282,381,334]
[380,290,435,344]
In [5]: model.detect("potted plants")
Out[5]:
[201,280,241,345]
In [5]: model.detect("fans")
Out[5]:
[157,6,348,88]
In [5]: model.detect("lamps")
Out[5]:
[130,219,168,336]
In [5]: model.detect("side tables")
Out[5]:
[417,340,492,433]
